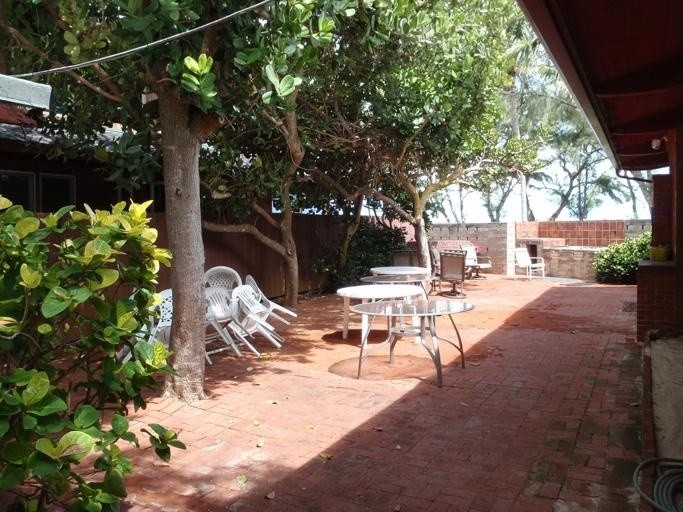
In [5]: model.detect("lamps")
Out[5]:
[651,136,668,150]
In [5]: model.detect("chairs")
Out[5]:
[116,266,298,365]
[513,247,545,281]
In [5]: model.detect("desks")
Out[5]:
[336,244,492,388]
